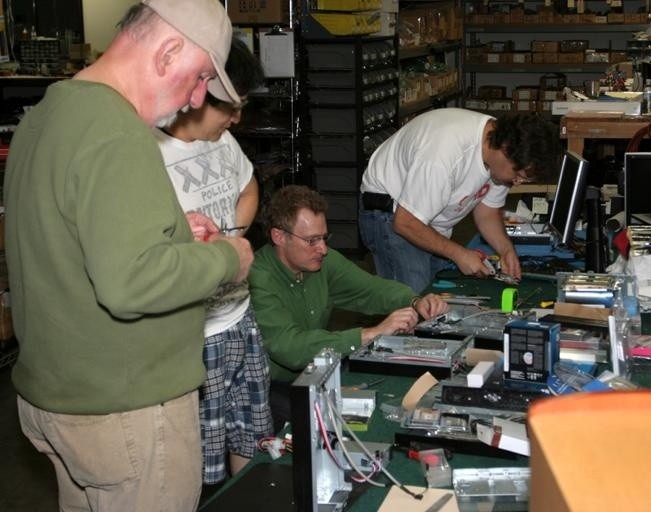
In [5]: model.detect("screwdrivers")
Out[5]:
[341,376,387,391]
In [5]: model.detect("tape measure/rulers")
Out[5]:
[501,287,518,313]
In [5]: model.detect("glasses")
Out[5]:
[274,223,332,245]
[210,97,250,113]
[512,170,536,183]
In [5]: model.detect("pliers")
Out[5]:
[398,447,439,466]
[433,279,470,289]
[473,250,519,285]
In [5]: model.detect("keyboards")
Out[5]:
[519,255,557,283]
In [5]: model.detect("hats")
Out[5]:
[139,1,243,108]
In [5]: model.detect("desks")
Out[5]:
[561,109,651,158]
[194,189,650,512]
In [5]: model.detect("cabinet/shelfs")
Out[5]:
[401,40,464,123]
[222,1,300,244]
[302,2,399,257]
[465,0,651,113]
[1,0,87,368]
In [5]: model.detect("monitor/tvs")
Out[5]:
[547,149,590,248]
[623,152,651,231]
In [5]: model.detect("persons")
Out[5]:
[148,37,277,485]
[0,0,256,512]
[357,106,565,304]
[245,183,453,433]
